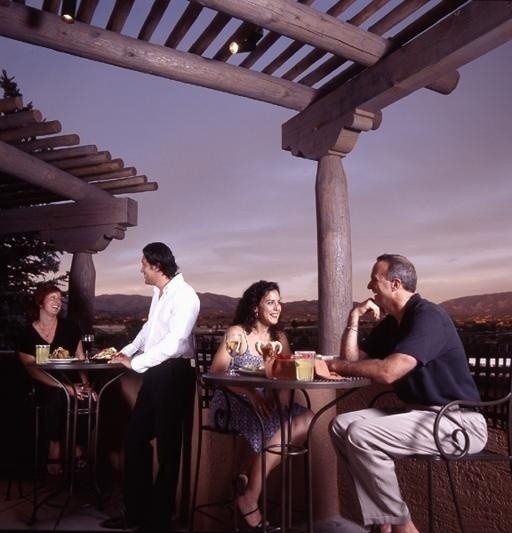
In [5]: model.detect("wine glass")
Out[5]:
[223,325,247,373]
[81,333,95,363]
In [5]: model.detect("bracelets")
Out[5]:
[343,325,358,332]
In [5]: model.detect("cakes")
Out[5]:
[50,347,70,359]
[93,346,117,359]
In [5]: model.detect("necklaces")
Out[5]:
[35,321,56,339]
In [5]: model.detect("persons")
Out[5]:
[14,281,98,476]
[325,252,490,533]
[207,278,316,530]
[97,239,201,531]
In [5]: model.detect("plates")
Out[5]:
[89,358,112,363]
[237,363,268,376]
[49,356,79,363]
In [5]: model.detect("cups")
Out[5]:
[35,343,51,365]
[294,350,316,382]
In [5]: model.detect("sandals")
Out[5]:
[235,473,249,495]
[46,443,88,477]
[236,498,269,527]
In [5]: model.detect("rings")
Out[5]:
[81,385,85,390]
[80,393,83,397]
[258,405,263,409]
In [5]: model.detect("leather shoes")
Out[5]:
[99,518,121,529]
